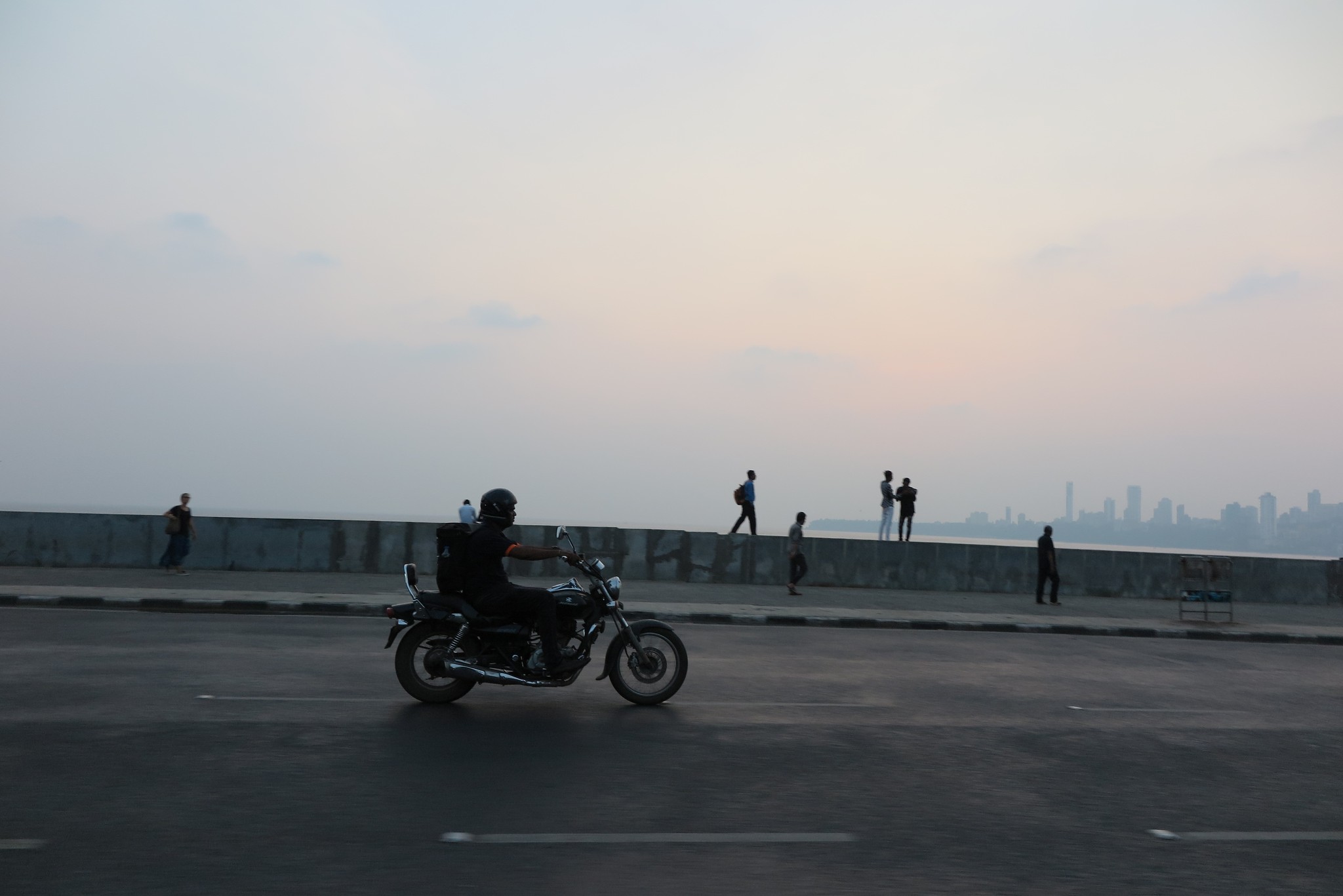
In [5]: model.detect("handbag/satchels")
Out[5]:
[165,505,182,534]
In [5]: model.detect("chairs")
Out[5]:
[1179,556,1234,623]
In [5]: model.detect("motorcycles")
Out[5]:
[384,526,691,707]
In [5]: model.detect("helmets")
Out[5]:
[480,489,517,512]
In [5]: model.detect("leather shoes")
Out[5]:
[550,657,591,674]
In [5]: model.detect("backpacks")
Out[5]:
[734,480,754,505]
[436,523,497,594]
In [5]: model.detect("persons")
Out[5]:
[787,512,808,595]
[877,470,917,541]
[1035,525,1063,606]
[158,493,197,576]
[730,470,759,536]
[458,499,478,524]
[460,489,592,671]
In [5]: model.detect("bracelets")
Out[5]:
[557,549,561,555]
